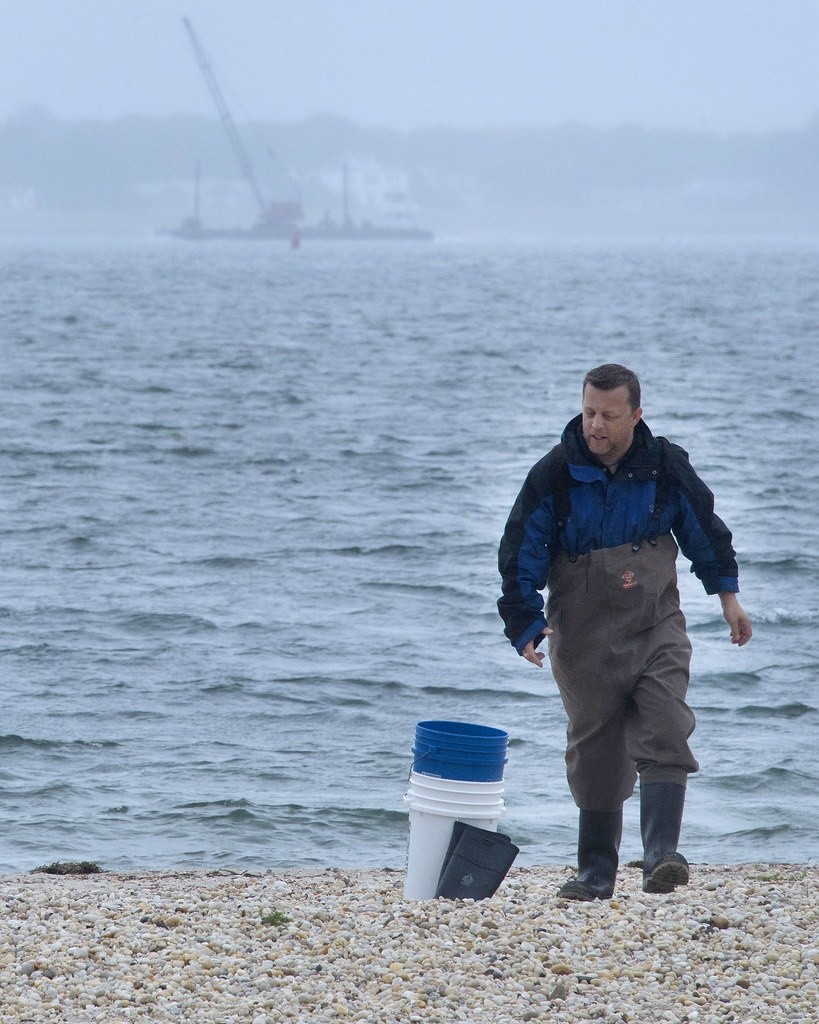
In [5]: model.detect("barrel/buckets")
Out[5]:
[411,720,509,782]
[403,768,506,900]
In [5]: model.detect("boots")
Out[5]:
[640,783,689,893]
[556,807,623,901]
[434,821,520,900]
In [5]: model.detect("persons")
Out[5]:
[497,363,753,901]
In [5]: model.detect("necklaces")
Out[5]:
[603,460,619,467]
